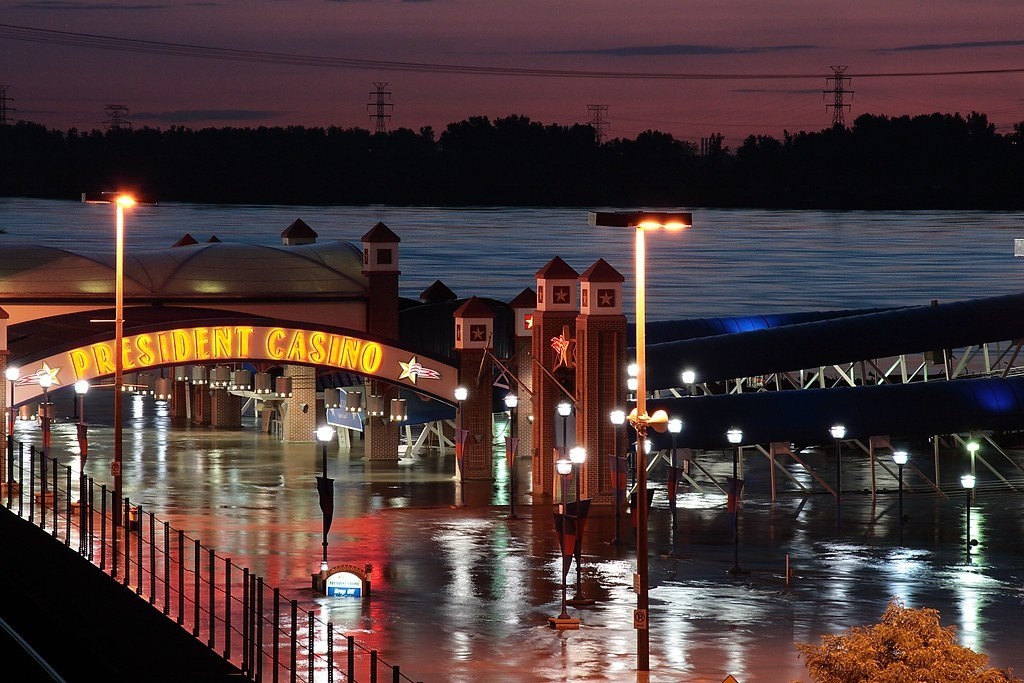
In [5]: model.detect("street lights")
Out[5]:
[569,445,587,600]
[726,425,745,575]
[554,457,572,619]
[114,194,137,526]
[664,417,683,558]
[888,451,910,523]
[556,401,572,505]
[314,425,334,565]
[591,209,694,672]
[608,409,626,546]
[504,391,519,519]
[827,421,848,530]
[74,379,90,458]
[3,366,20,437]
[38,373,53,449]
[453,385,469,508]
[959,473,977,564]
